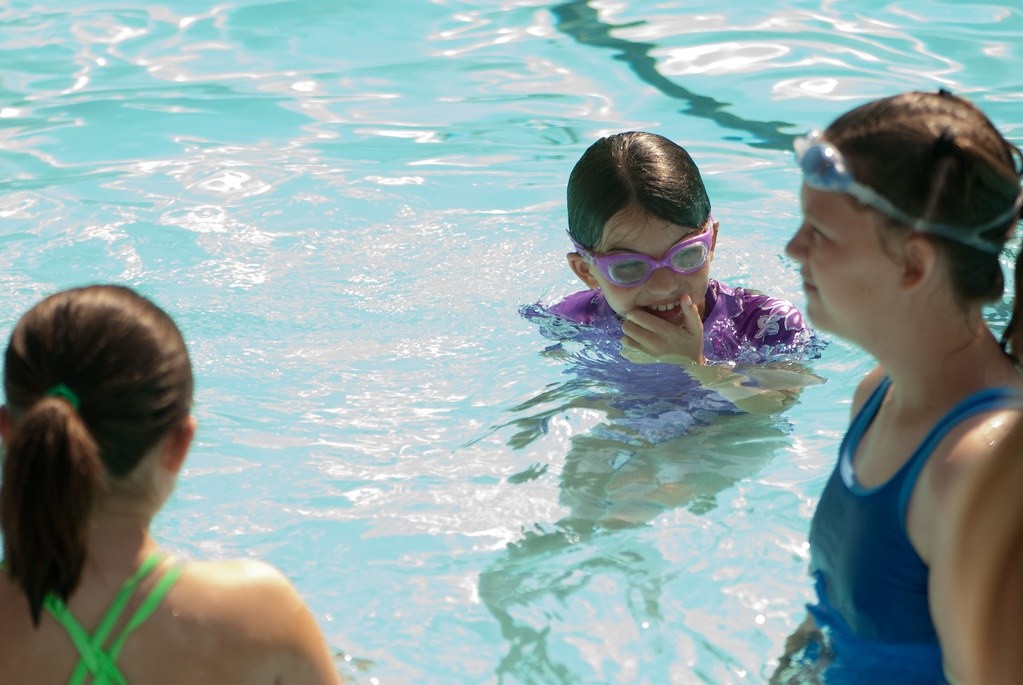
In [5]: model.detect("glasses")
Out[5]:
[566,215,715,287]
[794,125,997,254]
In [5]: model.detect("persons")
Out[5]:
[524,133,816,528]
[769,92,1022,685]
[0,285,344,685]
